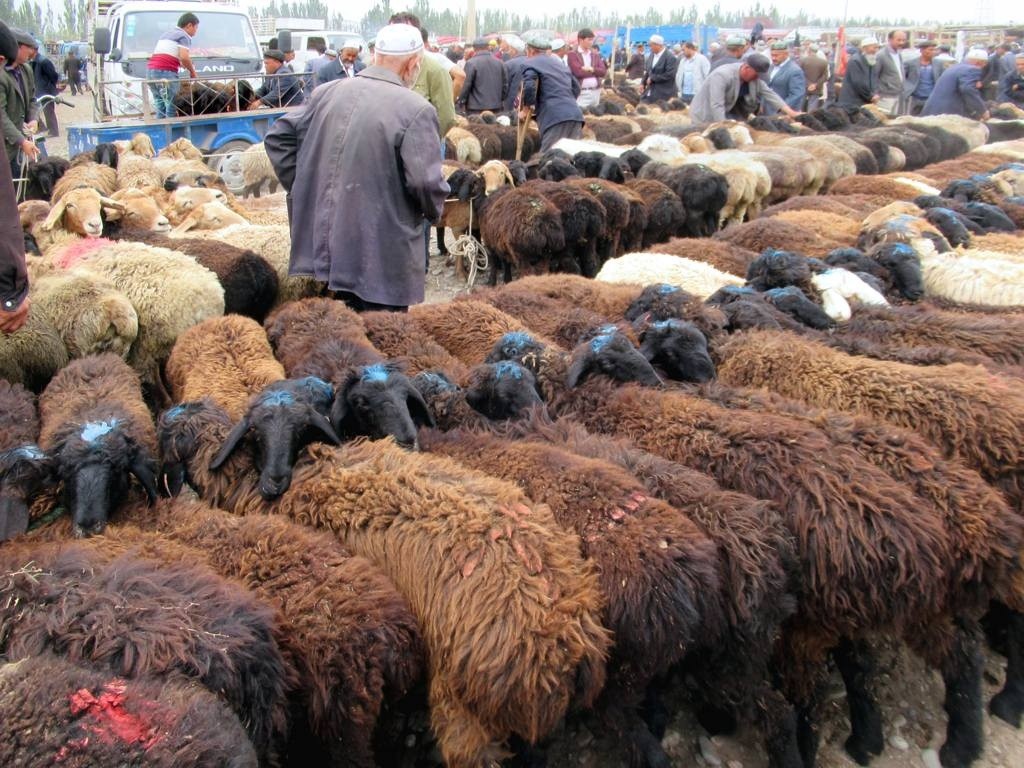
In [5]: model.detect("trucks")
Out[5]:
[87,0,260,122]
[57,42,88,81]
[248,18,372,84]
[593,24,718,70]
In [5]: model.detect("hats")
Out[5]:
[861,37,878,47]
[342,39,360,50]
[324,49,337,57]
[968,49,988,61]
[264,49,284,61]
[373,23,424,56]
[472,40,489,46]
[526,38,552,50]
[770,42,787,50]
[728,38,745,46]
[747,53,770,72]
[649,34,664,46]
[0,20,19,61]
[9,27,38,47]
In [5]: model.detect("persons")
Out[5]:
[0,20,40,203]
[592,45,608,68]
[567,28,606,107]
[521,36,585,153]
[747,30,958,116]
[421,28,528,114]
[248,49,304,110]
[29,44,59,136]
[608,35,746,105]
[283,37,376,97]
[921,49,990,119]
[978,42,1024,110]
[689,53,806,121]
[390,12,456,160]
[146,13,199,118]
[551,39,565,62]
[264,24,451,311]
[64,51,83,95]
[0,134,30,332]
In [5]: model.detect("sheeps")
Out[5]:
[0,89,1022,766]
